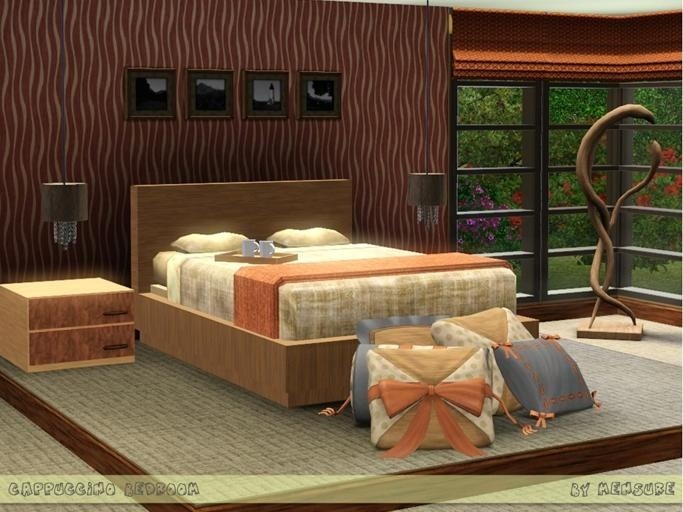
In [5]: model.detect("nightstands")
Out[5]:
[0,276,136,374]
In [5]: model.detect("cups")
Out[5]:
[259,240,275,258]
[241,239,258,258]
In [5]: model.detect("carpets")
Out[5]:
[0,397,145,512]
[0,339,682,471]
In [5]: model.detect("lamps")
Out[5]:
[39,0,89,251]
[406,0,448,227]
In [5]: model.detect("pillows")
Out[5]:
[169,231,251,253]
[265,226,351,247]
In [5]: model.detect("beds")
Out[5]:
[129,177,541,410]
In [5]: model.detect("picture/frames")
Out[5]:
[123,66,176,121]
[295,70,343,122]
[184,68,236,121]
[240,70,289,120]
